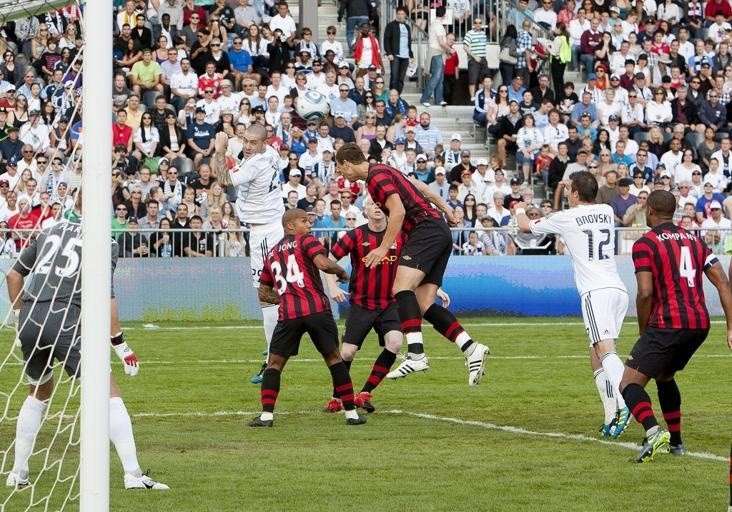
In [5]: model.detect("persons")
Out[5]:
[335,141,491,388]
[619,190,731,464]
[513,170,633,441]
[214,123,288,383]
[4,184,170,490]
[246,209,367,427]
[323,190,448,413]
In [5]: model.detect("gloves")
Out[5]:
[12,309,22,348]
[110,331,140,376]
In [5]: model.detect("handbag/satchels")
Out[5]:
[500,47,518,66]
[145,157,162,173]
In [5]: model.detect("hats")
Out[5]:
[339,62,348,68]
[280,111,521,187]
[577,6,722,209]
[197,79,264,119]
[1,80,74,187]
[127,216,139,224]
[481,215,493,222]
[368,64,376,70]
[158,157,169,165]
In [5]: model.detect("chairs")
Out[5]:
[672,27,709,45]
[141,89,162,109]
[633,130,730,165]
[171,157,195,175]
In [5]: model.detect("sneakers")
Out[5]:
[601,418,618,436]
[614,406,632,438]
[326,394,375,424]
[636,426,671,463]
[124,474,170,489]
[248,416,273,428]
[250,362,268,384]
[465,344,491,385]
[657,445,685,456]
[5,472,30,486]
[387,356,429,378]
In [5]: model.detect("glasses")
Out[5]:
[211,44,220,46]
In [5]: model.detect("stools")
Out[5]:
[471,118,570,212]
[578,61,585,81]
